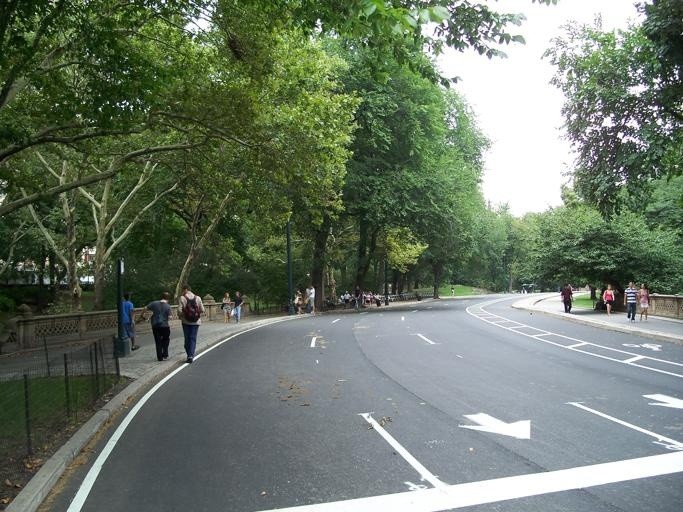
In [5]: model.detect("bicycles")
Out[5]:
[353,296,362,313]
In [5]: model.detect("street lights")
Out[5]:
[283,211,296,316]
[110,255,132,360]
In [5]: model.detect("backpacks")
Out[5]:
[182,294,200,322]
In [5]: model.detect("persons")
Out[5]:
[230,290,244,324]
[561,284,574,313]
[566,284,574,299]
[177,285,206,362]
[304,286,312,313]
[222,292,231,323]
[120,291,140,350]
[307,284,315,313]
[638,283,650,321]
[624,281,639,321]
[294,288,304,315]
[141,291,172,361]
[340,286,381,305]
[602,283,614,316]
